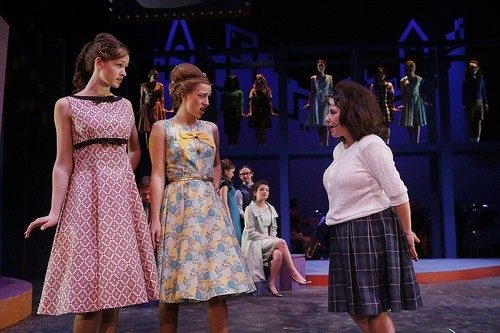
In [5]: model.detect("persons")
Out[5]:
[136,175,150,222]
[462,55,489,143]
[169,97,180,116]
[322,79,423,333]
[397,59,433,144]
[301,60,333,150]
[245,74,280,149]
[218,72,248,150]
[291,197,331,260]
[24,33,159,333]
[240,181,311,297]
[410,197,500,260]
[369,66,401,150]
[219,159,255,246]
[149,63,257,333]
[137,68,166,150]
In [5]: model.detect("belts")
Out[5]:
[169,175,214,183]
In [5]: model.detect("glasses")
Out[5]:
[240,172,251,175]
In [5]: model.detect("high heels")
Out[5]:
[267,287,282,297]
[291,275,312,286]
